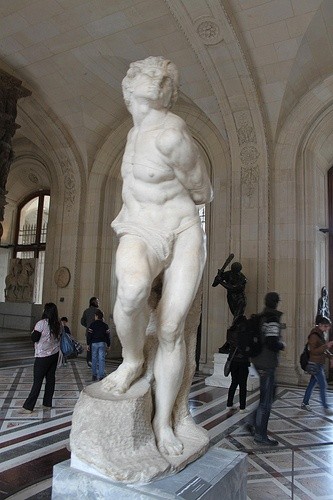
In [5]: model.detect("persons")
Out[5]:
[10,258,22,290]
[99,57,215,455]
[17,302,64,414]
[61,317,71,365]
[81,297,105,368]
[212,262,247,350]
[226,315,251,413]
[301,317,333,413]
[85,312,111,380]
[245,292,287,445]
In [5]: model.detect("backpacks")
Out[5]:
[237,312,275,357]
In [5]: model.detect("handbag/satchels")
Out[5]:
[60,333,72,357]
[73,340,84,353]
[300,350,310,371]
[224,360,230,377]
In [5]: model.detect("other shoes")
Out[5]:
[240,409,248,413]
[226,405,236,410]
[324,408,333,415]
[92,376,96,381]
[254,437,278,446]
[301,402,311,411]
[17,409,31,414]
[43,406,51,410]
